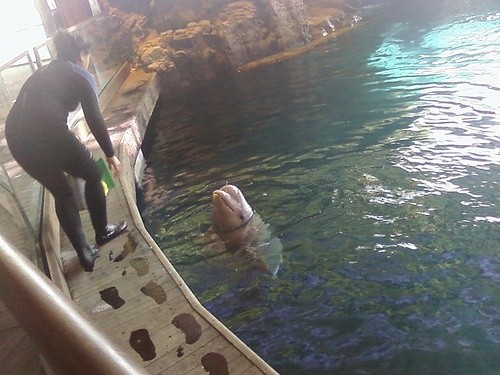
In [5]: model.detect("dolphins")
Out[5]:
[208,184,254,233]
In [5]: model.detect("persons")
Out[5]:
[5,29,127,271]
